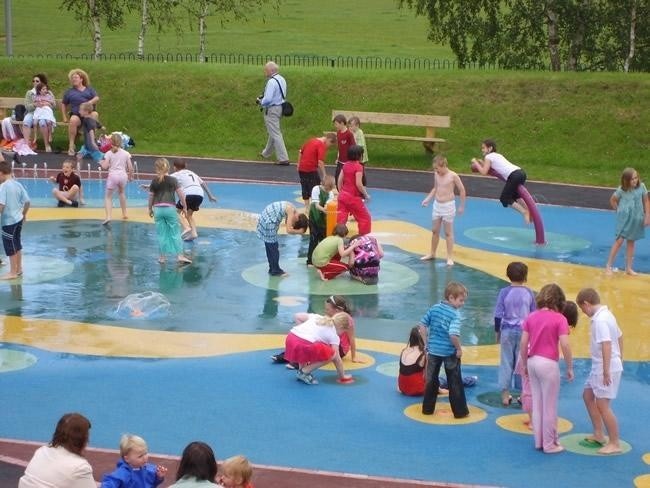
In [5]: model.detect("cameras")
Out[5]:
[256,95,264,104]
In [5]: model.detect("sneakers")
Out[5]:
[295,368,319,385]
[70,199,79,208]
[271,352,291,362]
[274,160,290,166]
[57,199,66,208]
[67,143,77,157]
[284,360,301,370]
[258,151,269,159]
[44,143,53,153]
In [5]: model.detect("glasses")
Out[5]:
[31,79,39,83]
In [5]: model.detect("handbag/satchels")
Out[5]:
[280,100,294,117]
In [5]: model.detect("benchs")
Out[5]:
[323,110,450,152]
[0,97,84,142]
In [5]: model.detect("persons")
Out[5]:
[139,158,217,240]
[347,235,383,279]
[512,301,578,429]
[306,175,336,267]
[493,262,536,407]
[348,118,369,199]
[309,224,352,281]
[164,442,222,488]
[97,133,135,224]
[255,201,309,276]
[296,133,336,217]
[471,141,533,226]
[47,159,86,208]
[0,152,16,267]
[606,168,650,276]
[0,160,30,280]
[31,83,54,144]
[331,114,356,192]
[148,157,192,264]
[398,326,478,396]
[421,155,465,266]
[17,414,101,488]
[257,62,291,166]
[23,74,56,152]
[270,296,367,369]
[418,281,469,418]
[78,102,106,130]
[519,283,574,454]
[281,310,353,386]
[576,288,624,453]
[214,455,254,488]
[61,69,100,157]
[336,145,371,235]
[99,433,168,488]
